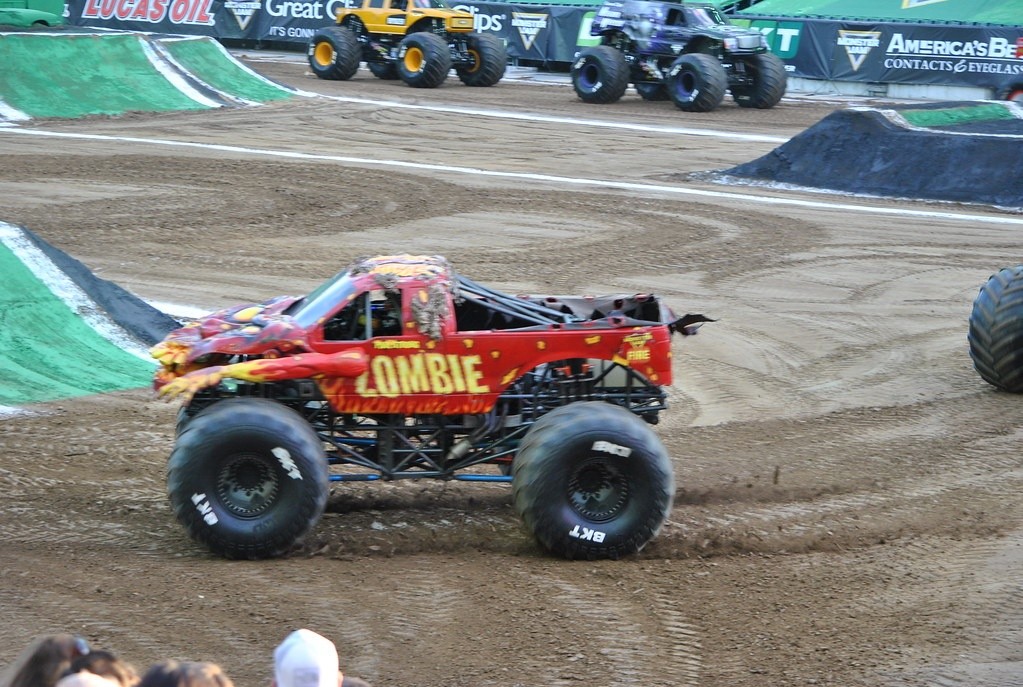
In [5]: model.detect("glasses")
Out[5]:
[73,637,89,662]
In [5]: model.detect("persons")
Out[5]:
[0,630,373,687]
[356,292,401,339]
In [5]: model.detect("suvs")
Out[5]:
[150,255,678,565]
[570,0,787,113]
[307,0,508,89]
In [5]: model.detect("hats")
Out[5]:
[273,628,338,687]
